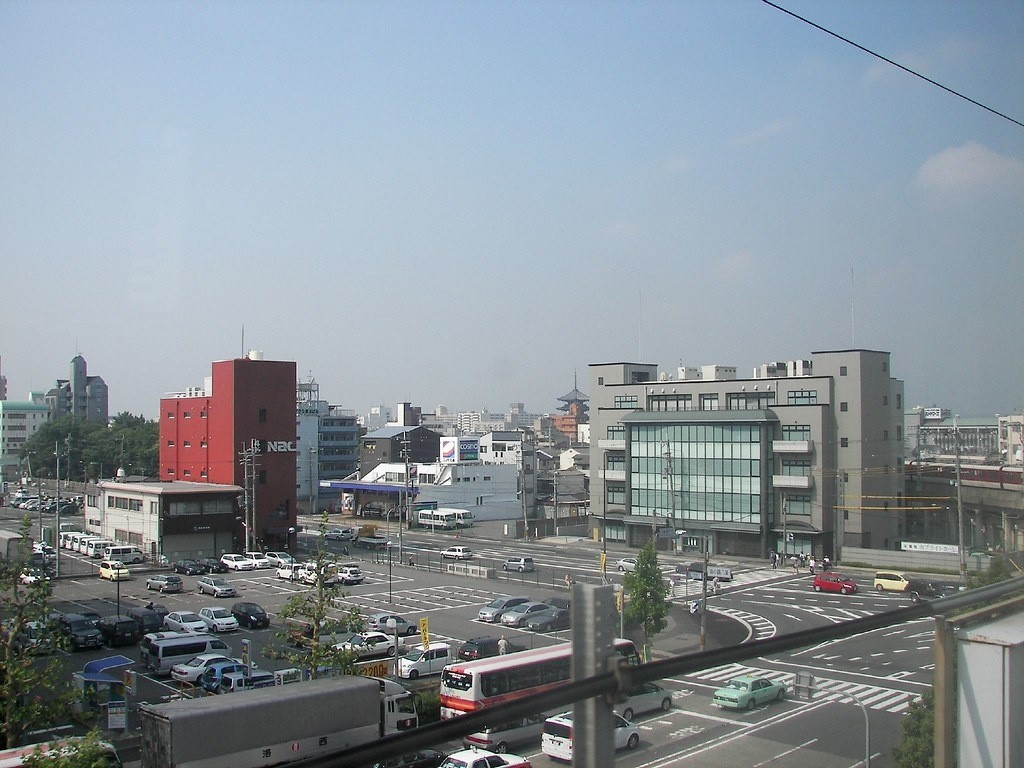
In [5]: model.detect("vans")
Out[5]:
[541,706,641,764]
[688,561,734,583]
[392,642,452,680]
[873,571,915,592]
[541,596,570,610]
[478,596,534,624]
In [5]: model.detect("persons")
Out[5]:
[770,550,783,569]
[800,551,830,573]
[410,554,414,566]
[498,636,508,655]
[344,545,349,555]
[146,602,153,609]
[713,577,719,595]
[565,572,573,589]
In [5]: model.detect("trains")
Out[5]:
[902,460,1024,484]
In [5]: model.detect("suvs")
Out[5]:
[502,556,536,573]
[440,545,473,561]
[457,635,525,663]
[907,578,953,604]
[322,527,352,541]
[364,613,417,637]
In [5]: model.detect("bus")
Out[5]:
[418,507,476,530]
[439,636,642,721]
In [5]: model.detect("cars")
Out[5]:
[812,573,858,595]
[331,631,406,663]
[12,529,367,698]
[615,557,648,573]
[524,608,570,634]
[609,681,672,721]
[500,602,559,628]
[371,747,448,768]
[462,709,555,754]
[436,745,533,768]
[712,673,788,712]
[8,485,85,517]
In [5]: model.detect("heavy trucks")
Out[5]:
[135,673,423,768]
